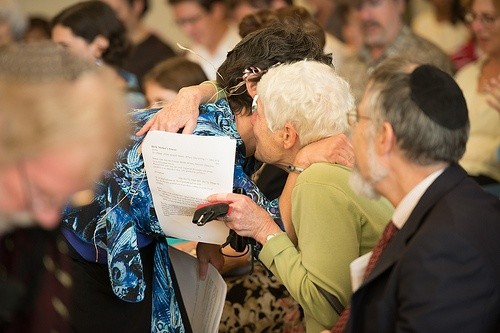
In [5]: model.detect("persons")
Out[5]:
[0,38,129,333]
[319,58,499,333]
[0,0,500,333]
[57,23,335,333]
[134,59,395,332]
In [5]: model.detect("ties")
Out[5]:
[331,220,399,333]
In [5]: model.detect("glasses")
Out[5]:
[347,110,390,127]
[465,13,500,23]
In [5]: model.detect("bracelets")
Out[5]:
[287,166,305,173]
[265,233,276,241]
